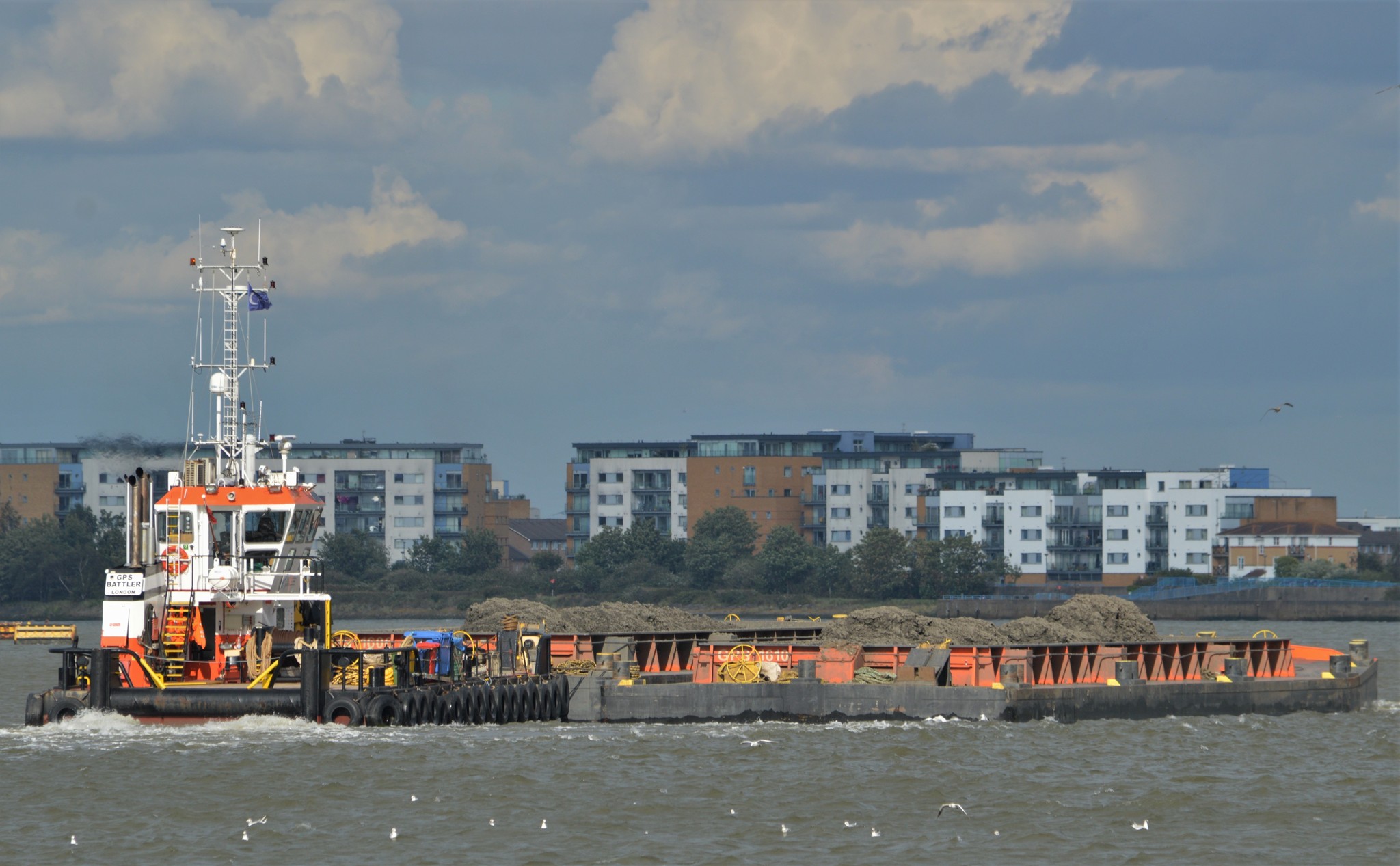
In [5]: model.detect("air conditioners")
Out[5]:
[185,457,216,487]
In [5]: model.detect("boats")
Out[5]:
[23,215,571,726]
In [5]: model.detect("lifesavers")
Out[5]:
[322,676,573,726]
[161,546,189,574]
[48,697,85,725]
[24,692,44,726]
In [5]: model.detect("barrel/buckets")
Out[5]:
[368,667,385,687]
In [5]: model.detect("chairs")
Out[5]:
[222,656,243,683]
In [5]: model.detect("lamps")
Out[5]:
[267,483,282,492]
[306,482,316,490]
[205,483,217,494]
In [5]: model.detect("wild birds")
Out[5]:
[489,819,495,826]
[389,828,398,838]
[781,825,791,833]
[844,821,857,828]
[1260,402,1293,421]
[541,819,547,829]
[410,795,418,801]
[993,831,999,835]
[71,835,78,845]
[247,816,267,827]
[242,831,248,841]
[871,827,881,837]
[739,740,780,747]
[1131,819,1150,831]
[730,809,735,814]
[935,803,968,819]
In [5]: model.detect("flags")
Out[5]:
[248,282,272,312]
[203,502,217,524]
